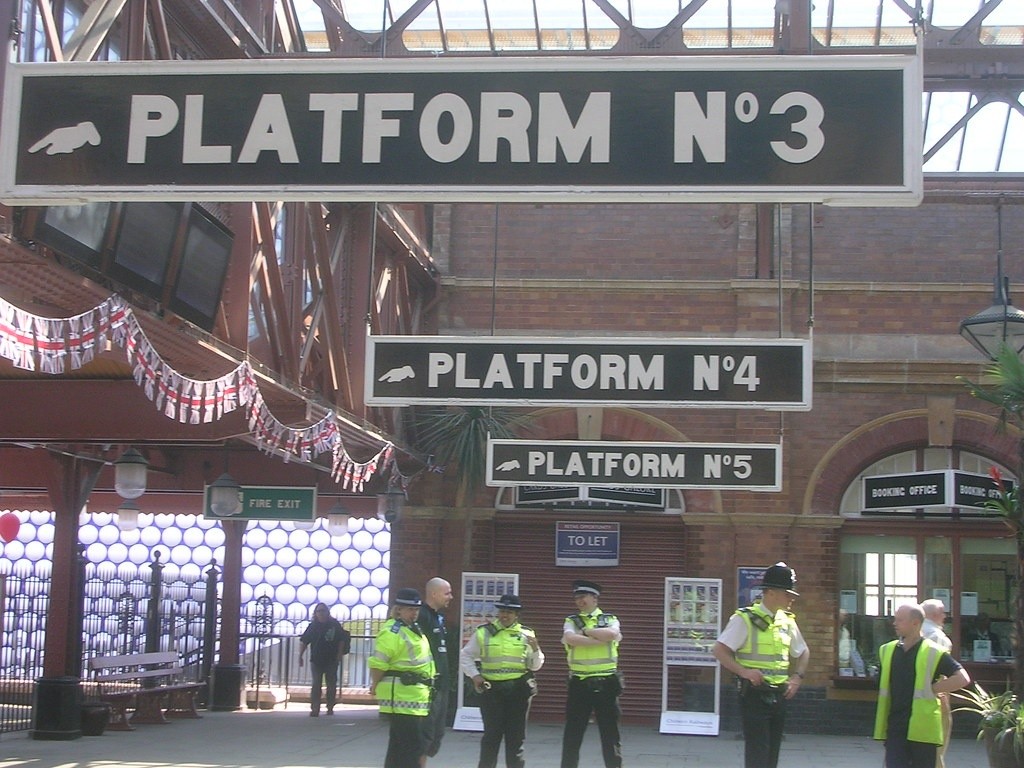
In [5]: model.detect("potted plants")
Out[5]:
[947,674,1024,768]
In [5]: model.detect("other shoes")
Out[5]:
[327,707,333,715]
[311,708,320,716]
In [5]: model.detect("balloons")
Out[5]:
[0,513,20,543]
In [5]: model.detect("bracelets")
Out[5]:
[794,672,804,679]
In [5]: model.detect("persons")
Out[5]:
[416,577,453,768]
[560,580,622,768]
[964,612,1001,655]
[921,598,953,768]
[461,595,545,768]
[712,562,810,768]
[367,587,440,768]
[873,603,970,768]
[298,603,346,717]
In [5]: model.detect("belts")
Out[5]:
[383,670,432,686]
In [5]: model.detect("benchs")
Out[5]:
[89,650,207,731]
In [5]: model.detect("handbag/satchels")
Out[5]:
[332,618,351,655]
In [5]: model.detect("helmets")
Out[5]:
[761,564,800,596]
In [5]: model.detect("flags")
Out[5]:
[0,293,424,502]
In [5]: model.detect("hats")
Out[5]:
[495,594,520,609]
[572,580,600,596]
[395,588,422,606]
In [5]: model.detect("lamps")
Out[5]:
[112,447,149,499]
[294,518,316,529]
[376,481,405,523]
[209,445,241,516]
[959,205,1024,365]
[327,497,349,536]
